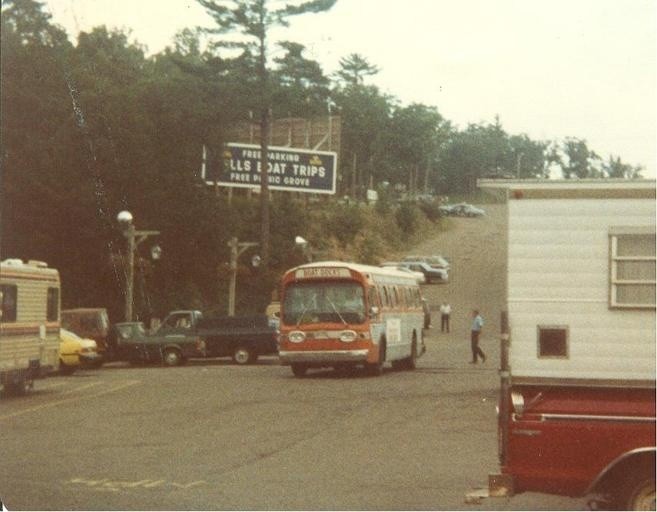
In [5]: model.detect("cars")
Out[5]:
[57,305,279,374]
[439,202,484,218]
[381,255,452,286]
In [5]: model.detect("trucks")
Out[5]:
[0,257,62,399]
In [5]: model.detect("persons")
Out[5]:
[439,299,453,334]
[467,310,487,364]
[340,288,364,314]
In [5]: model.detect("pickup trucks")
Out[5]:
[462,362,656,511]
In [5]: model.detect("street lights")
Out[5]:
[120,225,161,316]
[225,236,260,317]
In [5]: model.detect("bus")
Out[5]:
[276,260,430,377]
